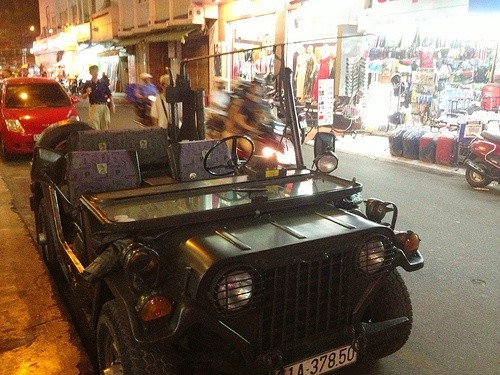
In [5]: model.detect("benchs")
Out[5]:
[69,127,175,193]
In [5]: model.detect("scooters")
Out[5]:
[204,113,287,162]
[454,130,500,188]
[131,95,158,128]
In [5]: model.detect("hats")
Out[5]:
[159,74,176,87]
[139,72,153,81]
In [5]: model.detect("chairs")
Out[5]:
[65,149,142,238]
[174,138,234,183]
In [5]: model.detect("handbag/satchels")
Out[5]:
[167,122,181,139]
[370,43,500,126]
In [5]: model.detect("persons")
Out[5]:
[222,81,272,162]
[150,75,182,129]
[214,45,222,75]
[101,72,110,88]
[133,73,159,118]
[82,65,116,129]
[294,44,336,106]
[253,50,268,80]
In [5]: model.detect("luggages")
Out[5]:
[390,126,458,166]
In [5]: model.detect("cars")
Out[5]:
[0,77,82,156]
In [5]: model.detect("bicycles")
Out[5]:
[296,99,353,137]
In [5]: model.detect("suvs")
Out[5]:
[29,118,426,375]
